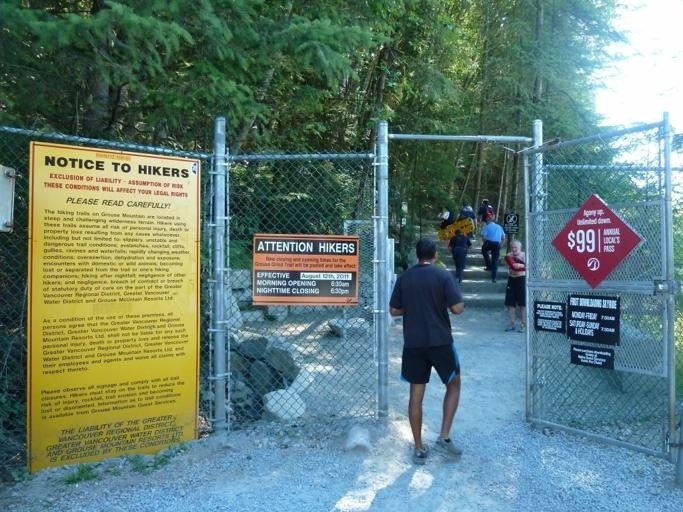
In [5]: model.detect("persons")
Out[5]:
[386,233,466,464]
[435,198,525,333]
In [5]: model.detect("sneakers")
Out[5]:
[505,326,516,332]
[411,442,431,466]
[433,437,463,461]
[519,324,525,332]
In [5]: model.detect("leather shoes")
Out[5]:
[492,278,496,282]
[484,266,489,271]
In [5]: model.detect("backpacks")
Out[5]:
[483,205,495,220]
